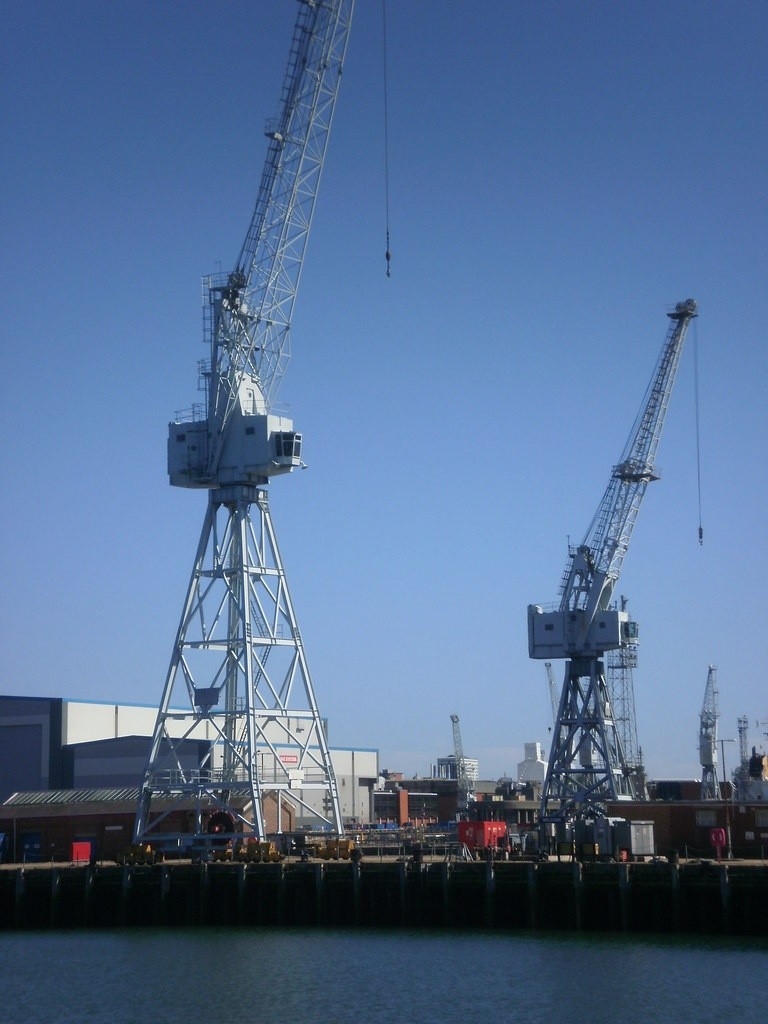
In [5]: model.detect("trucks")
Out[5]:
[458,822,527,860]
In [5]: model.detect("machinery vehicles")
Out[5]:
[222,840,283,863]
[316,840,354,861]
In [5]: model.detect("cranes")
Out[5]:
[126,0,391,854]
[697,662,723,800]
[527,296,707,823]
[544,660,561,725]
[449,712,471,811]
